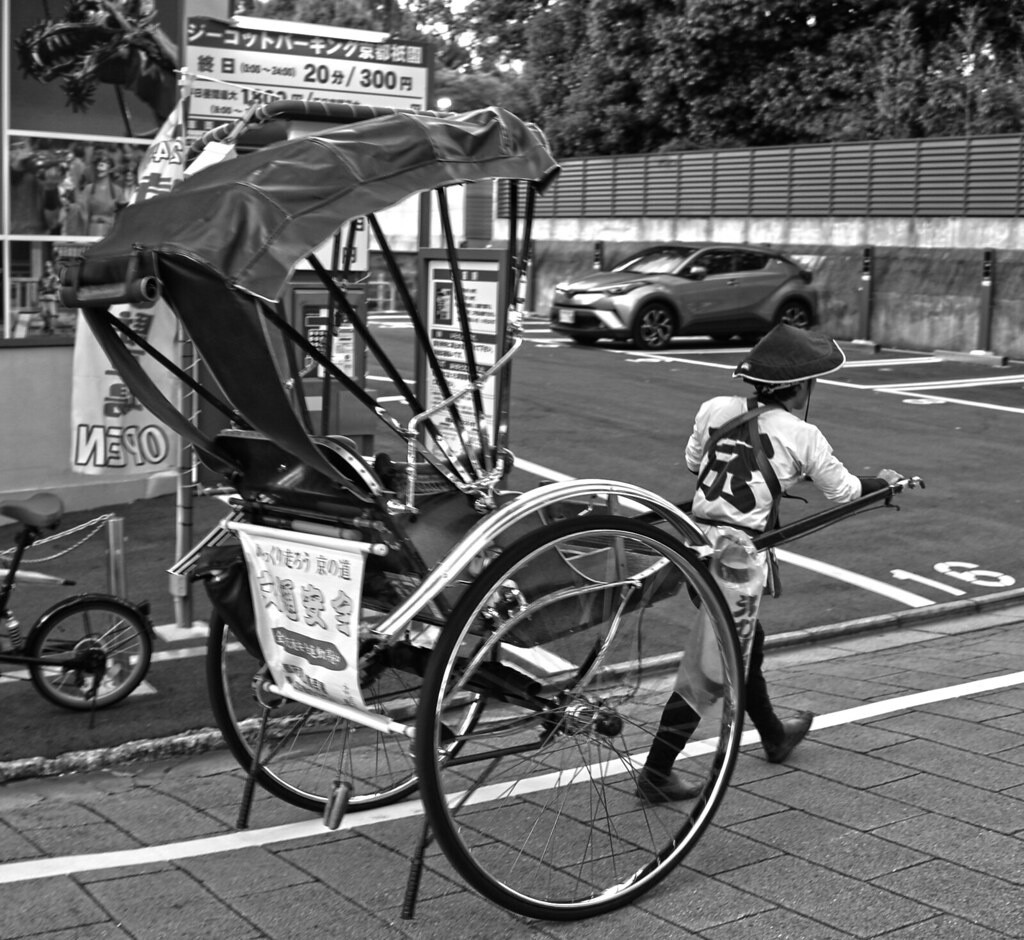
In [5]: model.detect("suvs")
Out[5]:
[550,243,822,351]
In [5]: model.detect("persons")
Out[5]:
[37,261,62,335]
[638,324,907,804]
[14,139,128,239]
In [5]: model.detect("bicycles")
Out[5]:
[1,490,157,733]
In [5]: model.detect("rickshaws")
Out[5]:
[54,104,927,925]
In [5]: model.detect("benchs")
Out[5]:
[206,427,683,646]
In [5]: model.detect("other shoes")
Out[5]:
[636,768,703,801]
[766,712,813,762]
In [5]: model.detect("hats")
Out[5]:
[732,322,845,383]
